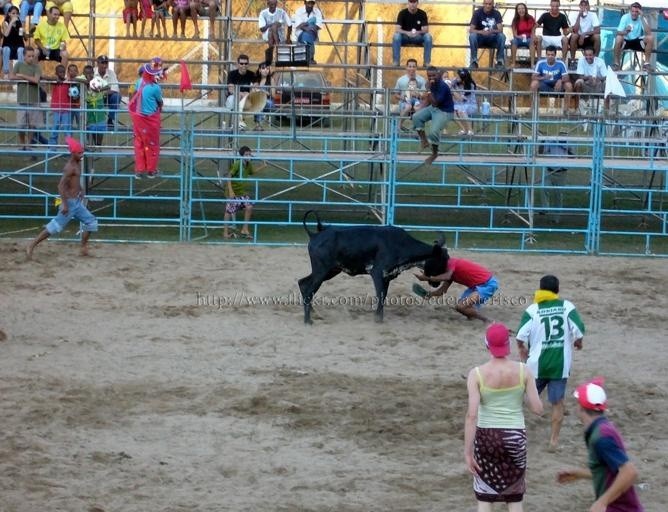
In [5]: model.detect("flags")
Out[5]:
[179,60,192,94]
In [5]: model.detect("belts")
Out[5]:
[547,168,565,172]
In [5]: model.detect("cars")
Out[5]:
[266,69,333,129]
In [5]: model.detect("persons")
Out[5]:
[573,49,611,118]
[1,0,117,151]
[463,321,545,512]
[152,0,171,39]
[554,381,642,512]
[222,146,268,239]
[23,137,100,257]
[515,275,586,450]
[290,0,323,65]
[221,55,255,134]
[393,59,478,156]
[509,3,538,69]
[169,1,190,40]
[567,0,601,70]
[127,56,167,178]
[609,3,655,72]
[525,46,571,116]
[252,62,282,132]
[259,1,291,49]
[123,0,139,37]
[391,0,433,68]
[189,0,222,41]
[468,0,506,69]
[411,257,496,322]
[138,0,156,38]
[533,127,576,221]
[530,1,569,61]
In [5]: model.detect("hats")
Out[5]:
[97,55,109,63]
[573,379,606,411]
[66,138,82,152]
[145,58,163,73]
[485,323,509,357]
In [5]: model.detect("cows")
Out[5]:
[298,210,450,324]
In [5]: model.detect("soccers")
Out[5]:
[69,86,79,97]
[90,77,104,91]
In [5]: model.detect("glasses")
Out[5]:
[240,63,248,65]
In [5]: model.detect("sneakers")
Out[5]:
[135,170,161,179]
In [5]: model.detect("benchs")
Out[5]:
[2,0,666,173]
